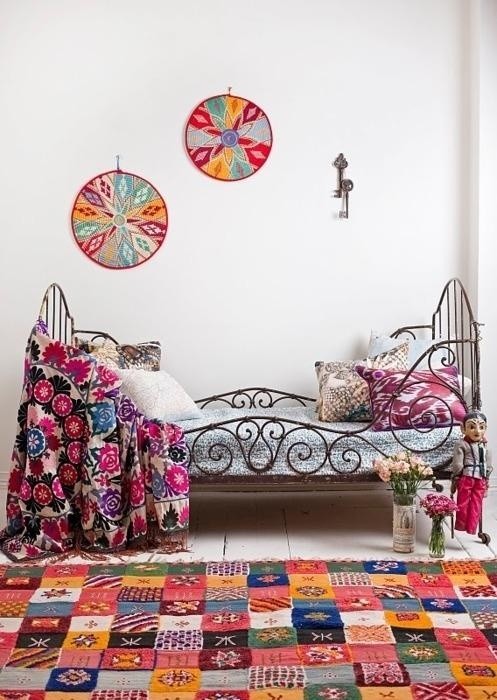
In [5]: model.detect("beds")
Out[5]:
[32,278,491,545]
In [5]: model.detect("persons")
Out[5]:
[450,412,492,533]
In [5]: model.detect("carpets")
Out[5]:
[0,556,496,700]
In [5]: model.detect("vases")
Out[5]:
[427,522,448,557]
[392,493,417,553]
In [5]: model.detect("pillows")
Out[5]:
[353,362,468,432]
[71,335,160,369]
[87,336,140,369]
[314,341,410,424]
[113,365,204,423]
[365,326,449,371]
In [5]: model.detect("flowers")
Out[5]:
[368,451,436,506]
[419,492,458,554]
